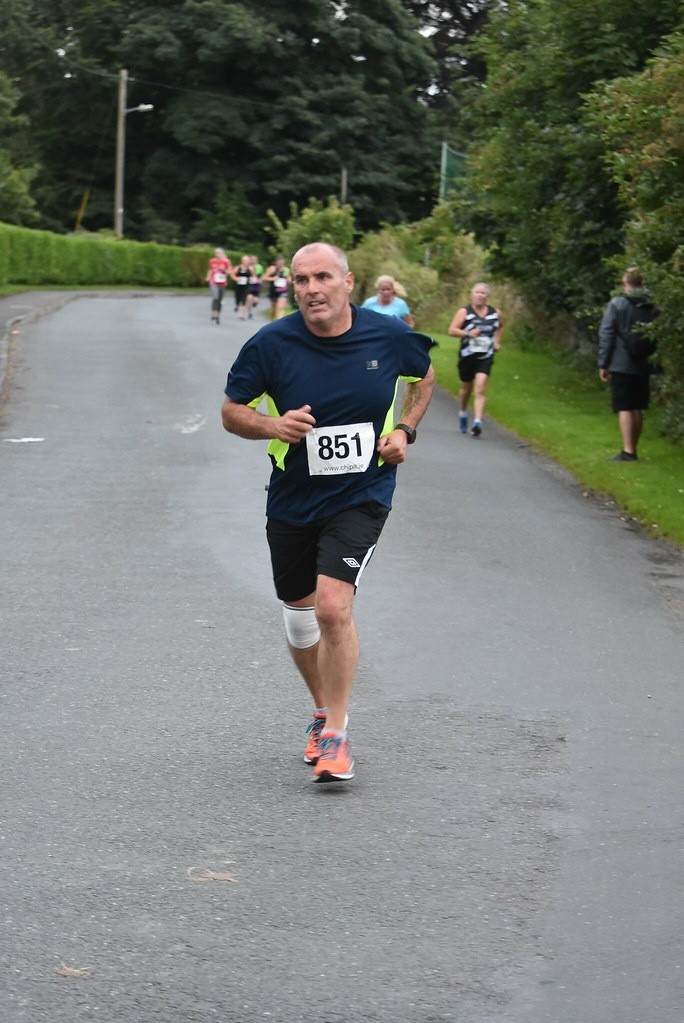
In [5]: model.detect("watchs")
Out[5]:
[395,424,416,444]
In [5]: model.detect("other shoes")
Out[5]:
[613,450,637,462]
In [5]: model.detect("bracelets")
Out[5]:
[465,331,468,337]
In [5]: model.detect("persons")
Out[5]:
[231,253,264,319]
[260,256,293,325]
[449,283,503,436]
[359,275,414,329]
[205,247,234,323]
[221,242,434,781]
[597,267,660,462]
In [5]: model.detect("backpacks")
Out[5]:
[615,293,660,356]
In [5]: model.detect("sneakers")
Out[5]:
[312,732,355,784]
[303,711,349,766]
[459,417,467,433]
[471,423,482,437]
